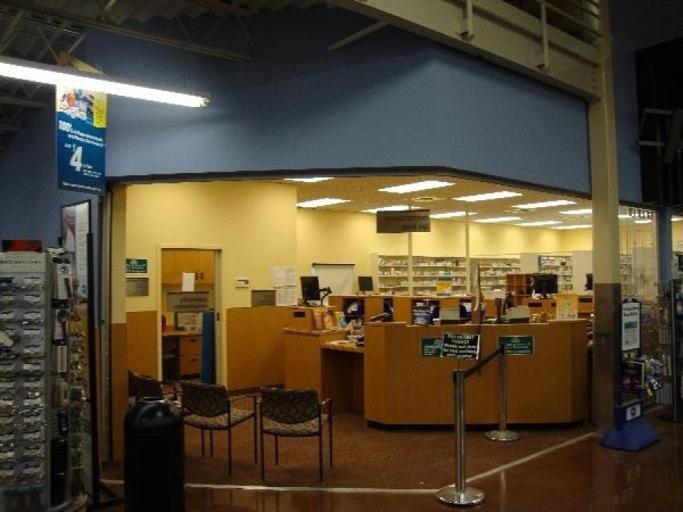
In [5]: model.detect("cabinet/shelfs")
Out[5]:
[538,252,574,293]
[378,254,521,300]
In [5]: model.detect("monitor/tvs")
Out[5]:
[300,276,320,307]
[251,289,276,307]
[359,276,373,291]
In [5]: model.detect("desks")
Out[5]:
[280,309,600,431]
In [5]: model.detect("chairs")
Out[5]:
[125,366,334,484]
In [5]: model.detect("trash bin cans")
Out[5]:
[123,395,188,512]
